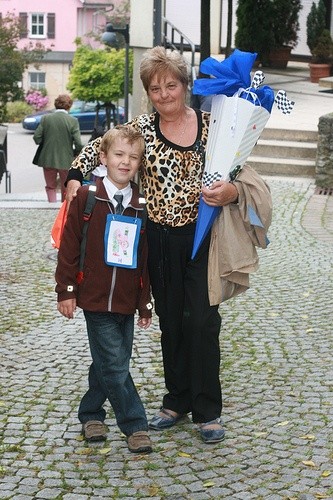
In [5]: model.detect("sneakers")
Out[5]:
[127,430,152,453]
[82,419,106,441]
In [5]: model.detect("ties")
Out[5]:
[114,194,124,215]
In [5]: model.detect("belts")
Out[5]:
[146,221,195,234]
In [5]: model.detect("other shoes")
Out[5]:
[149,407,187,430]
[199,418,225,443]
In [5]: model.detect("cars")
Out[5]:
[22,100,126,134]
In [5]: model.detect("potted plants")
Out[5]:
[307,0,333,83]
[235,0,303,68]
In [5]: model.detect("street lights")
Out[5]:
[102,23,130,123]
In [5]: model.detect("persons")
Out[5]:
[54,126,152,453]
[34,94,81,203]
[63,47,239,443]
